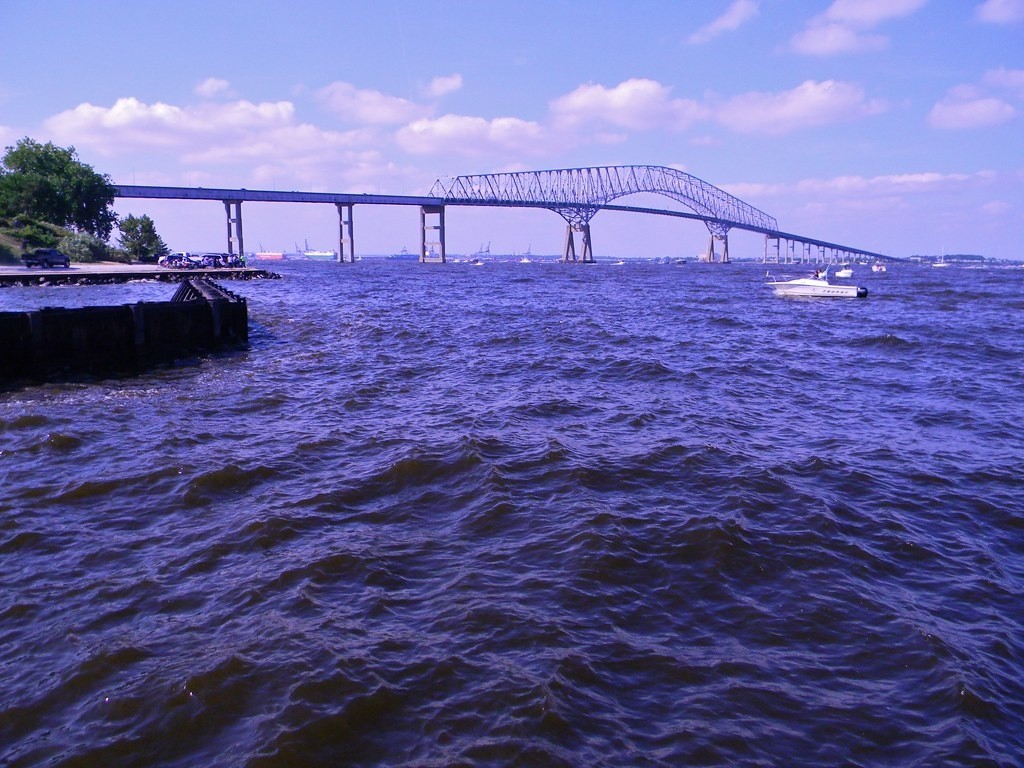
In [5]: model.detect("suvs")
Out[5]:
[200,252,238,267]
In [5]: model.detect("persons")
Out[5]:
[164,254,246,269]
[814,269,823,280]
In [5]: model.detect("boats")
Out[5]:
[871,257,886,272]
[658,256,669,264]
[256,242,284,260]
[838,261,850,265]
[294,238,338,260]
[763,258,869,298]
[860,261,867,265]
[470,241,556,265]
[675,257,687,265]
[424,240,470,263]
[388,246,420,260]
[611,262,625,266]
[834,265,854,277]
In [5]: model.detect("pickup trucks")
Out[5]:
[158,251,212,269]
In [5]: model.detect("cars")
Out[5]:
[20,247,70,269]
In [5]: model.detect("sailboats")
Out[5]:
[932,242,950,269]
